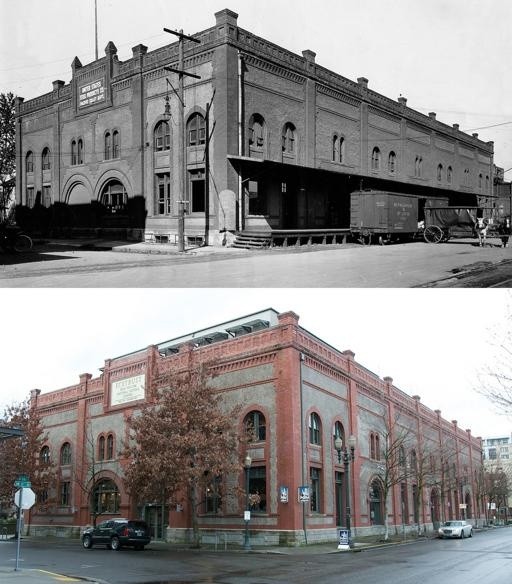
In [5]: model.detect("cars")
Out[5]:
[80,516,152,552]
[437,519,474,539]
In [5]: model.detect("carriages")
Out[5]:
[423,204,511,249]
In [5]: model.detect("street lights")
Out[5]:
[333,434,358,550]
[241,450,253,551]
